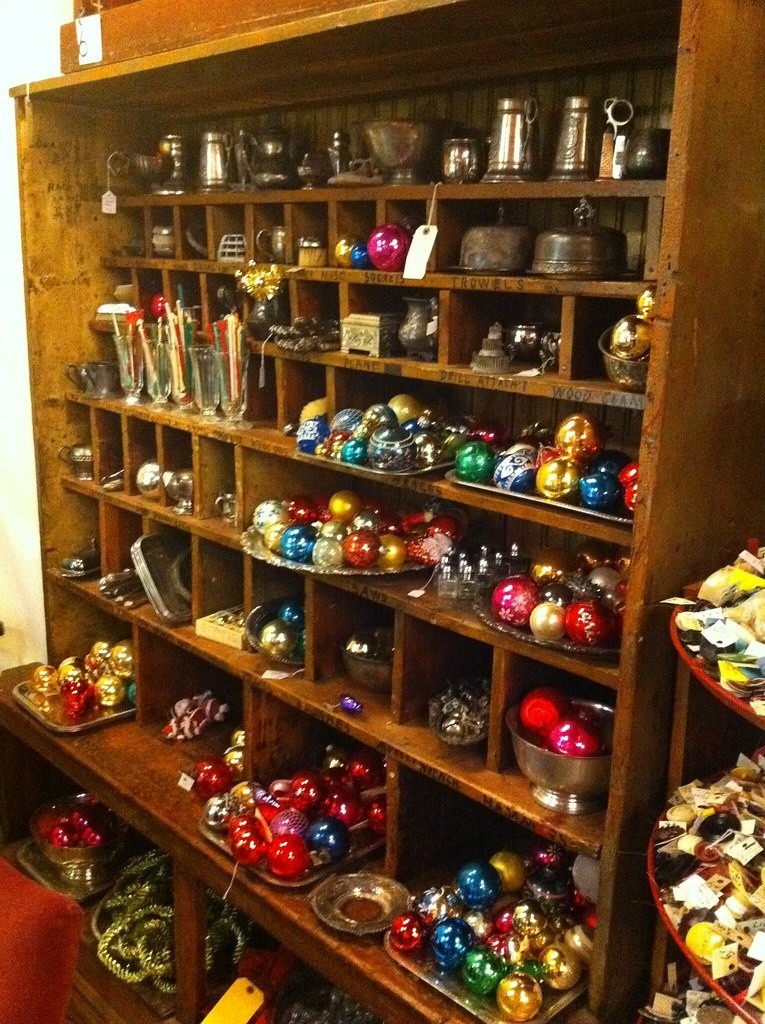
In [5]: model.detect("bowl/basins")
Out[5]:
[340,643,393,692]
[30,795,128,881]
[506,699,614,815]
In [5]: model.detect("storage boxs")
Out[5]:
[340,309,408,360]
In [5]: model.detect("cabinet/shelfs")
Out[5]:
[0,0,764,1024]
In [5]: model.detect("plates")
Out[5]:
[472,583,620,662]
[311,870,413,935]
[245,596,304,665]
[237,524,433,578]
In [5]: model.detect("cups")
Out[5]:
[113,323,254,432]
